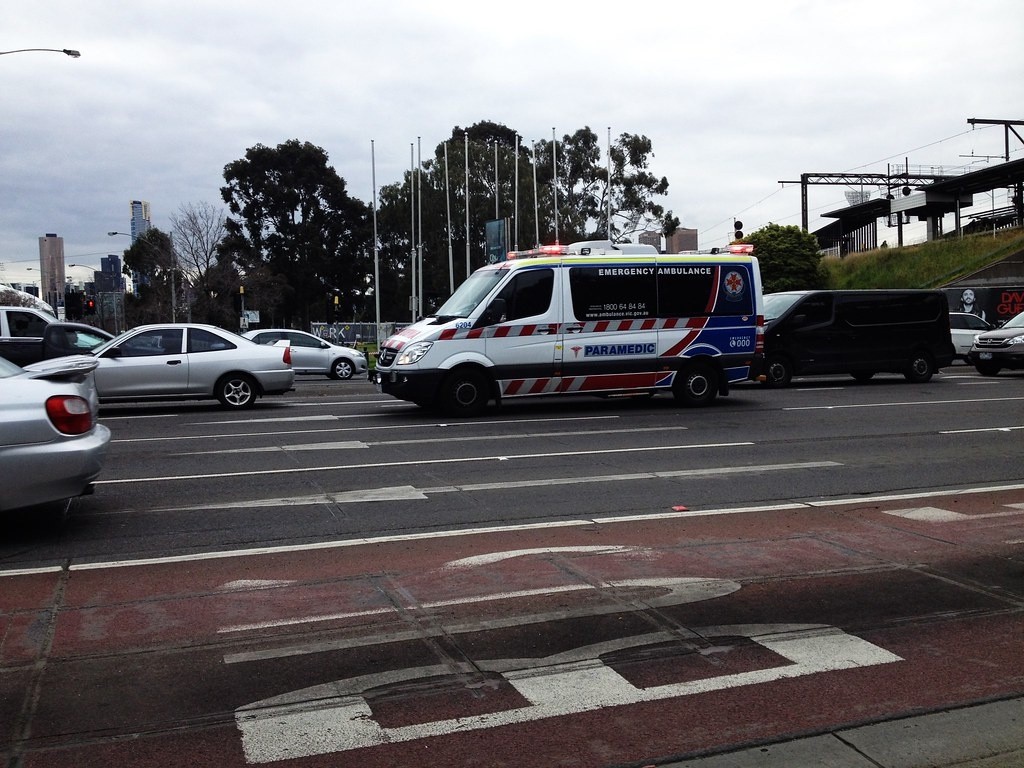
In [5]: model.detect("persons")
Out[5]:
[881,240,887,249]
[954,288,996,329]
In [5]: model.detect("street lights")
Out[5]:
[24,229,176,339]
[0,48,82,59]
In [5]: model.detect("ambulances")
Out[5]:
[372,239,767,420]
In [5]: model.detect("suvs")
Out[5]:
[949,308,1024,379]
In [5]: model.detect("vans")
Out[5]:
[750,288,955,384]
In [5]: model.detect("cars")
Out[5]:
[0,307,114,515]
[25,323,295,410]
[243,329,367,382]
